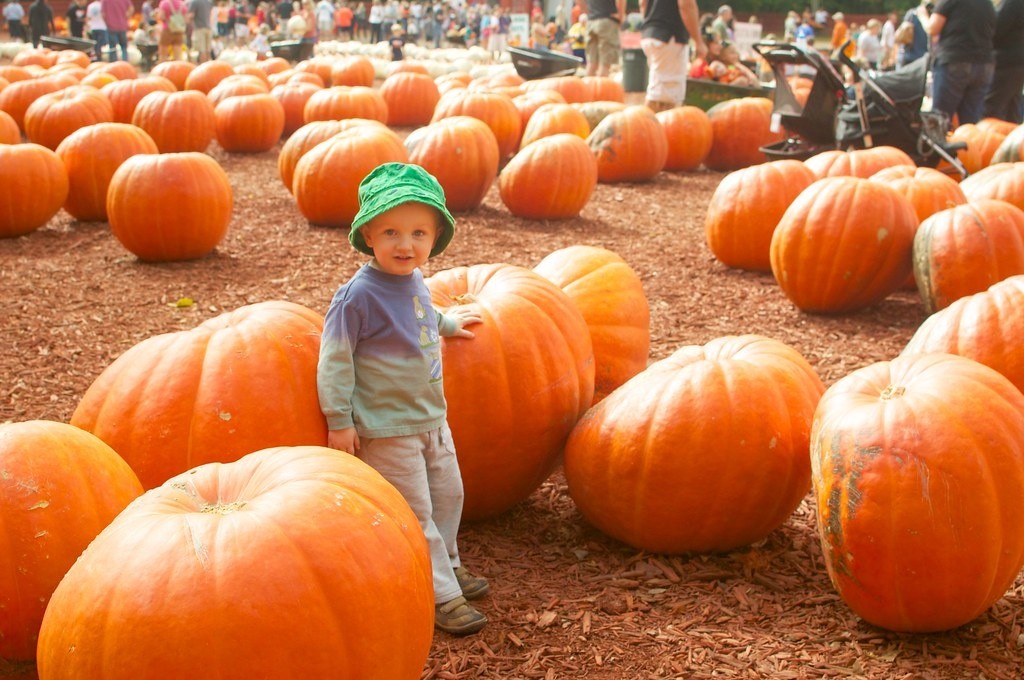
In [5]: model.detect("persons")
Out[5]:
[0,0,1024,178]
[316,164,491,634]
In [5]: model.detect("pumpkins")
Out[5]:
[0,40,1024,680]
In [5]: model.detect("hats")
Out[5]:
[348,162,455,259]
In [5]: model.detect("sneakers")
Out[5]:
[435,596,488,636]
[452,567,489,600]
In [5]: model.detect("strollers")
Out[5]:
[752,39,969,178]
